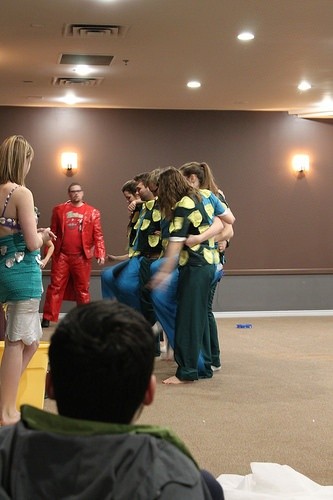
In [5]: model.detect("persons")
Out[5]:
[101,161,236,384]
[0,299,225,500]
[41,183,105,329]
[0,135,58,426]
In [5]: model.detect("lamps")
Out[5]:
[292,154,311,178]
[61,152,78,170]
